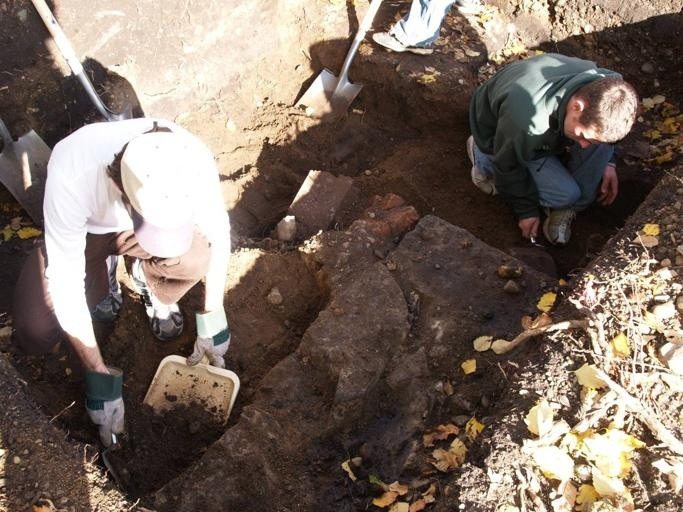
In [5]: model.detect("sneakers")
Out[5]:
[372,27,436,57]
[93,254,125,324]
[127,259,184,342]
[464,136,499,197]
[540,203,577,248]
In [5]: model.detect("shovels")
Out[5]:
[141,353,240,429]
[30,0,134,126]
[102,432,132,492]
[292,0,383,124]
[0,118,52,231]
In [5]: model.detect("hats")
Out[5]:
[122,126,211,257]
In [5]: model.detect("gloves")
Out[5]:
[184,307,232,368]
[84,365,127,448]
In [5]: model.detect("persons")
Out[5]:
[9,115,232,446]
[465,50,641,247]
[369,0,482,58]
